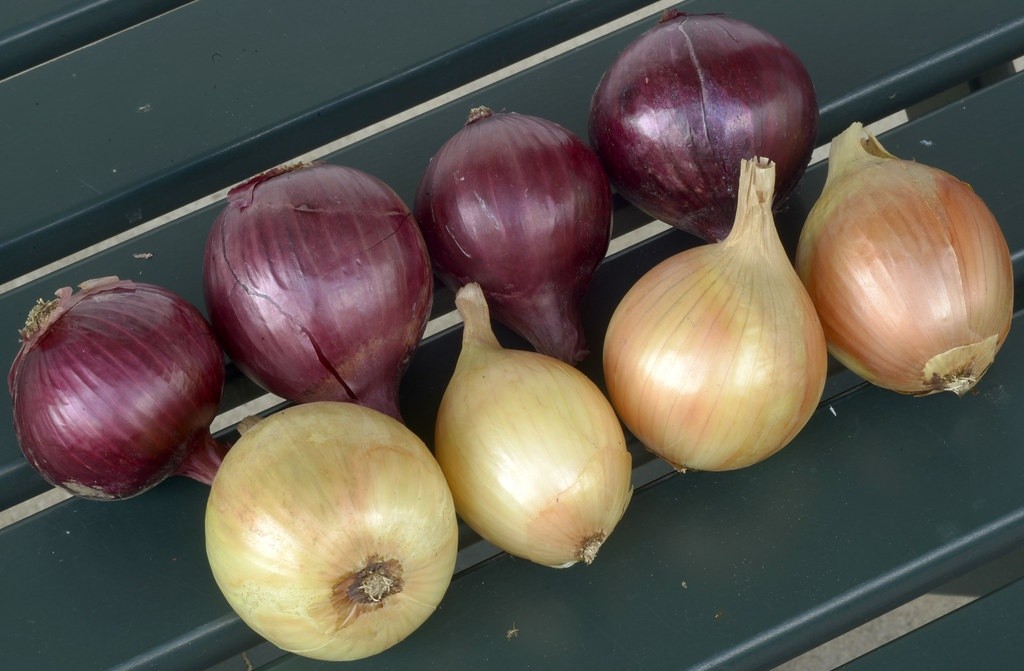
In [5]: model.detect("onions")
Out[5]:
[7,6,1015,662]
